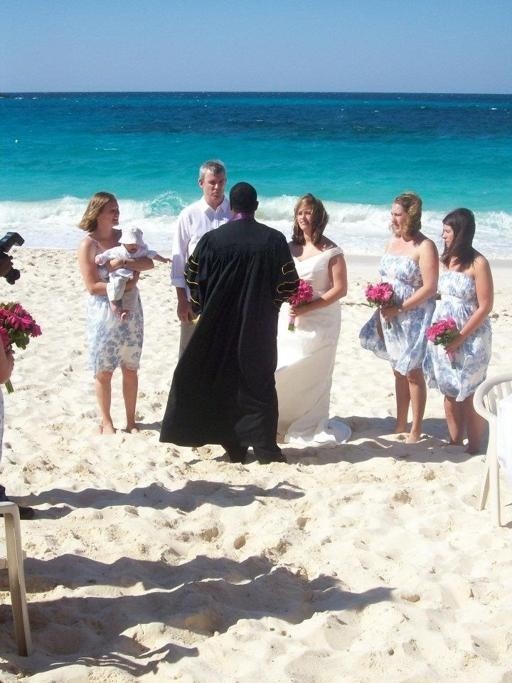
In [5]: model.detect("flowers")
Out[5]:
[365,282,394,329]
[425,318,458,369]
[0,301,42,393]
[288,279,313,331]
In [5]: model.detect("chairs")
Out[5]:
[473,373,512,527]
[0,501,31,658]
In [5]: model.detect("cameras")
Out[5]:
[1,232,24,285]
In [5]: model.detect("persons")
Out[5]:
[0,252,14,278]
[94,227,173,324]
[169,161,235,363]
[77,191,155,436]
[0,301,43,520]
[274,193,353,450]
[358,191,439,445]
[158,182,288,464]
[421,207,494,455]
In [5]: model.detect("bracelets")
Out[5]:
[123,259,128,270]
